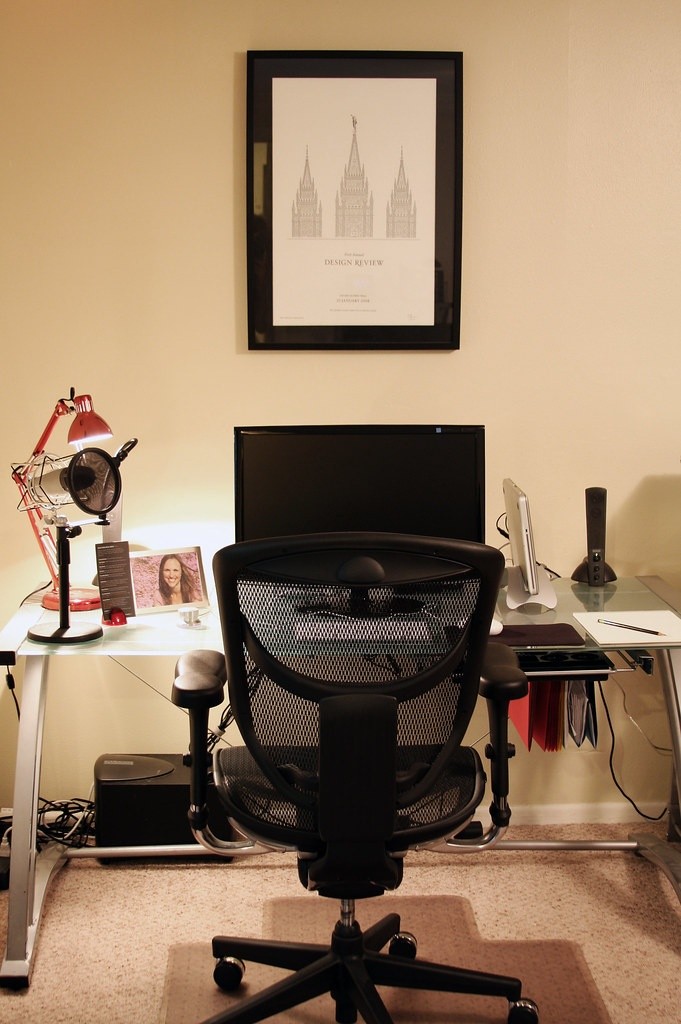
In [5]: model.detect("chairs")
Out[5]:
[170,533,540,1024]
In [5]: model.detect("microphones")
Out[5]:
[32,465,96,496]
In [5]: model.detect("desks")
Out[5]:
[0,576,680,989]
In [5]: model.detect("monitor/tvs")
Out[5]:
[235,424,486,619]
[503,478,558,609]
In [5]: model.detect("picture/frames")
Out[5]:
[129,546,210,616]
[246,50,463,350]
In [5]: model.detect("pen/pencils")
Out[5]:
[598,618,666,637]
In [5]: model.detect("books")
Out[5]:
[573,610,681,647]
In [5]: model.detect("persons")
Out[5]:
[152,555,200,606]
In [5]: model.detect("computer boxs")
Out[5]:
[94,752,234,865]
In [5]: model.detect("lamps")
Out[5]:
[11,387,114,611]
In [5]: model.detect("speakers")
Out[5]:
[571,487,617,587]
